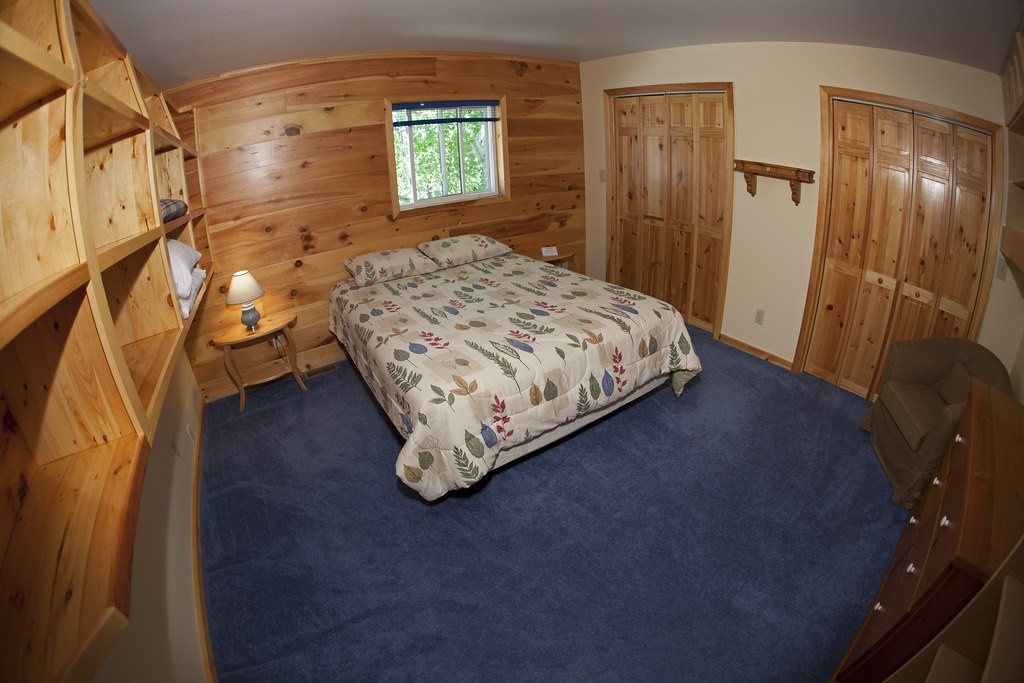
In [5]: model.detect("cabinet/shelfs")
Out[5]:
[827,21,1024,683]
[0,0,217,682]
[614,91,727,334]
[804,100,994,404]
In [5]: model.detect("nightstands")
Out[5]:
[213,313,307,413]
[535,249,580,274]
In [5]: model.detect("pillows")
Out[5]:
[343,247,440,287]
[417,234,513,269]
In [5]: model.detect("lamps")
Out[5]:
[226,270,265,331]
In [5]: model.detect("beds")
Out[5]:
[327,251,703,503]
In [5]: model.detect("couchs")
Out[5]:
[859,337,1014,512]
